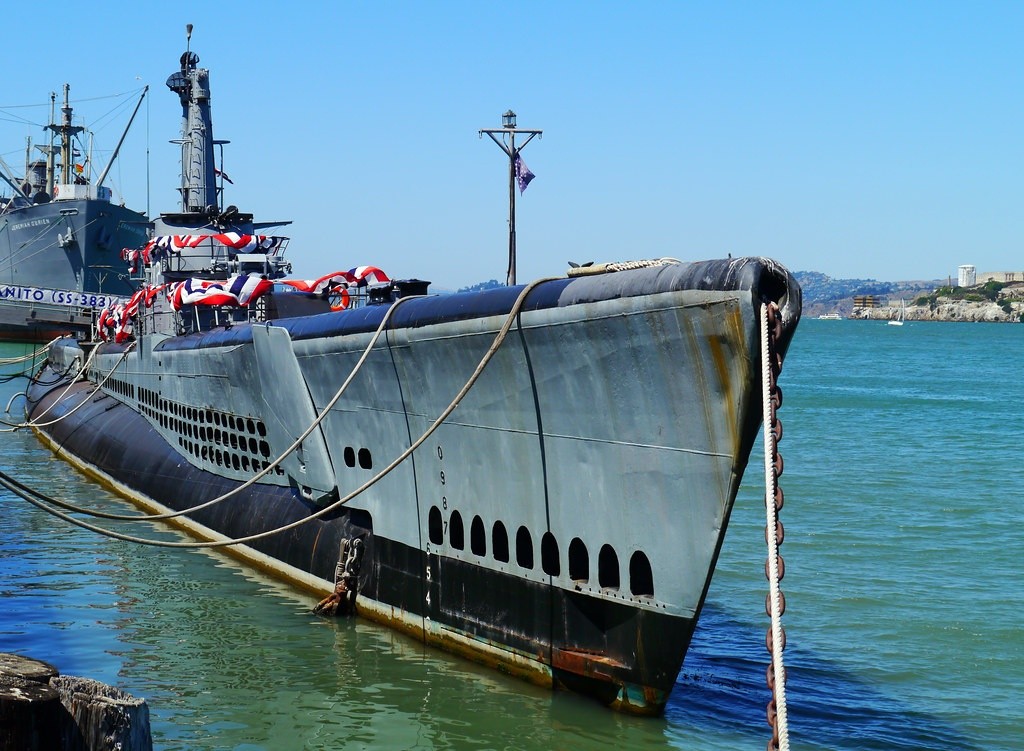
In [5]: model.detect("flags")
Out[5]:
[76,164,83,173]
[513,148,535,197]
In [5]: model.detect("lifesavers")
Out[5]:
[328,287,350,311]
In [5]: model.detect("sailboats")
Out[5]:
[888,299,905,326]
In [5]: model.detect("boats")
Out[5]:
[24,20,803,716]
[0,81,149,342]
[818,312,843,321]
